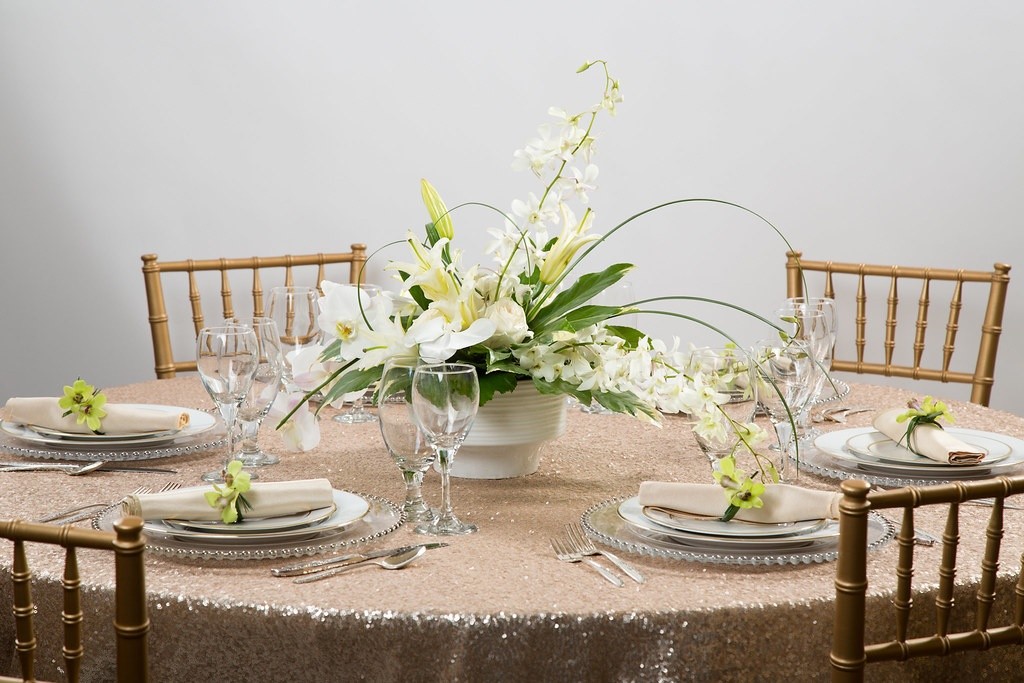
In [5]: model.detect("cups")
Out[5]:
[263,286,323,396]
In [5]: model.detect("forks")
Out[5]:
[563,522,644,585]
[548,535,624,587]
[37,482,182,526]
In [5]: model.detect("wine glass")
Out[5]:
[378,359,439,523]
[222,316,284,465]
[757,296,837,484]
[411,364,480,537]
[195,325,261,484]
[684,348,758,484]
[322,279,384,424]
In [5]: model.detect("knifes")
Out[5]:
[270,542,450,575]
[0,461,178,476]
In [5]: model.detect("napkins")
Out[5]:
[5,397,191,435]
[121,477,333,519]
[638,480,843,524]
[872,409,985,466]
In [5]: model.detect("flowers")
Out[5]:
[896,398,951,455]
[204,460,254,525]
[60,376,108,437]
[716,458,764,519]
[274,60,843,484]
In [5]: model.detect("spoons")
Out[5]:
[0,458,111,475]
[293,546,426,584]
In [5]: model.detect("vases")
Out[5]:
[432,380,567,478]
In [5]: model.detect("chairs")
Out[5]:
[142,242,367,381]
[784,247,1010,407]
[837,477,1024,681]
[0,516,152,683]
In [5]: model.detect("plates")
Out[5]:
[845,432,1011,465]
[0,408,230,461]
[580,494,896,566]
[0,403,217,449]
[164,502,336,528]
[27,421,164,438]
[812,379,849,405]
[640,506,827,536]
[92,488,406,559]
[785,430,949,487]
[813,427,1024,471]
[141,489,371,539]
[617,497,839,550]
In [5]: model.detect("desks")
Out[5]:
[0,362,1024,681]
